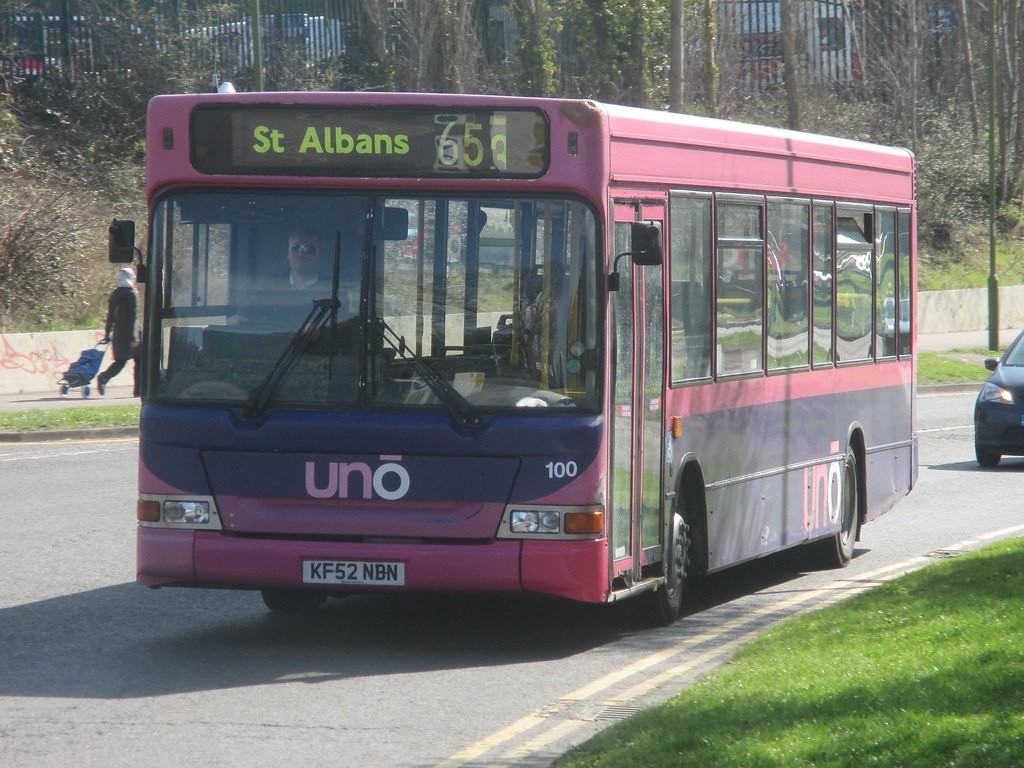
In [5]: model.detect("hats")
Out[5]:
[121,268,135,279]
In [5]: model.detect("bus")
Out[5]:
[108,87,920,628]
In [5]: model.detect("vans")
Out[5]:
[6,15,163,91]
[882,297,911,356]
[710,1,865,96]
[173,16,343,72]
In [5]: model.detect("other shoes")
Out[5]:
[98,375,104,395]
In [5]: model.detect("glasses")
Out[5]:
[290,243,317,254]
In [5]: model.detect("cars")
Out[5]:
[974,331,1024,466]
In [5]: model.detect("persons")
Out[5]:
[226,225,351,355]
[97,268,142,397]
[520,278,556,373]
